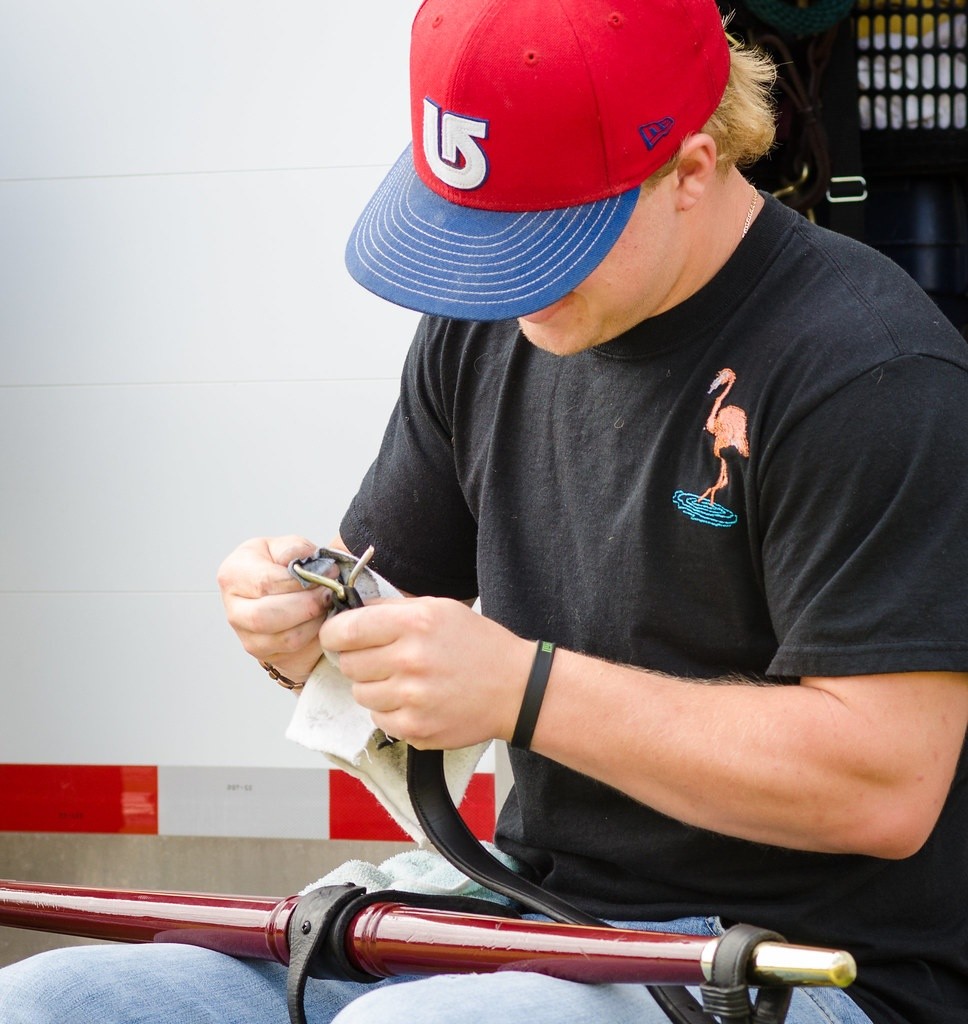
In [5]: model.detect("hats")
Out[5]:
[346,0,730,322]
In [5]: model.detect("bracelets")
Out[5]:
[510,640,555,752]
[257,660,306,690]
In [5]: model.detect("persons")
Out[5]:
[0,0,968,1024]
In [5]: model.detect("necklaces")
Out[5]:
[742,184,759,238]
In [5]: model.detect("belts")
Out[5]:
[286,544,791,1024]
